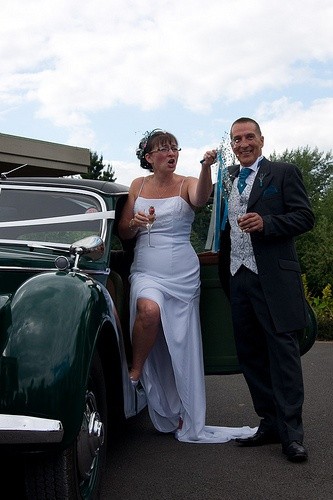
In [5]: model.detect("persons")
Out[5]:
[217,117,316,462]
[116,128,260,443]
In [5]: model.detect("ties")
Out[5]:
[237,167,253,196]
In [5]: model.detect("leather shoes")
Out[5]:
[235,429,284,445]
[282,439,308,460]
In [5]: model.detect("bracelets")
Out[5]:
[128,219,139,233]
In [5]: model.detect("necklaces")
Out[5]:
[153,179,165,198]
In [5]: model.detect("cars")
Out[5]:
[0,177,318,500]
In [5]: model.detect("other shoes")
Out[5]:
[129,370,142,388]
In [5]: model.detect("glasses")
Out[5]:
[148,146,181,154]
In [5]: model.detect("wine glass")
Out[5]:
[237,202,253,254]
[143,205,156,248]
